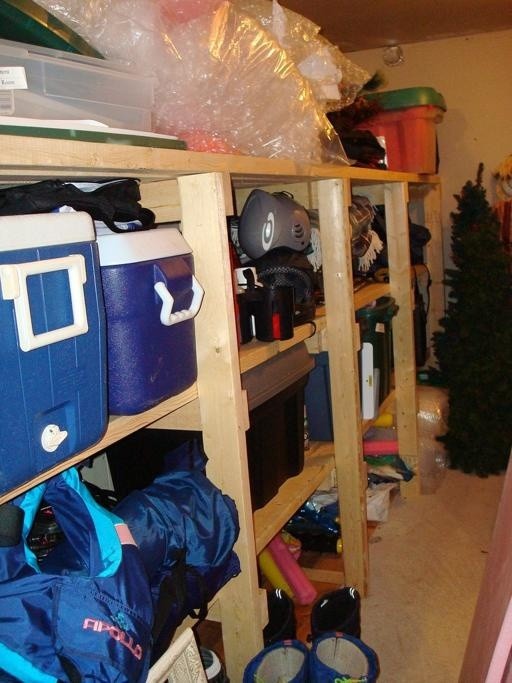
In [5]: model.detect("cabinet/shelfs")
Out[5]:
[0,135,445,683]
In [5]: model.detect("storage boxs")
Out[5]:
[329,85,449,173]
[0,211,109,498]
[94,226,205,415]
[1,36,156,133]
[239,341,316,515]
[355,295,400,408]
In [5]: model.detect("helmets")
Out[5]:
[240,188,311,258]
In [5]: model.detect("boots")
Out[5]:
[239,585,382,683]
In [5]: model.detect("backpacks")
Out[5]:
[0,176,156,233]
